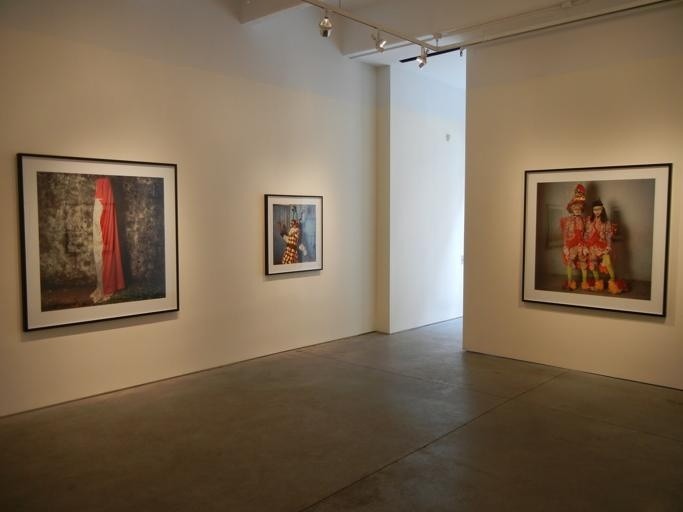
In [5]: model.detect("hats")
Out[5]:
[567,184,586,213]
[593,199,603,206]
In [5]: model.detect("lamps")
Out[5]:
[304,0,439,70]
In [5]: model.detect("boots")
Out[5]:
[568,281,621,294]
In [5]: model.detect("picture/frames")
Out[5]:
[264,193,323,275]
[521,163,672,318]
[16,152,179,332]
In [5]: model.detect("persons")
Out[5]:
[583,200,624,295]
[559,184,589,290]
[282,210,303,267]
